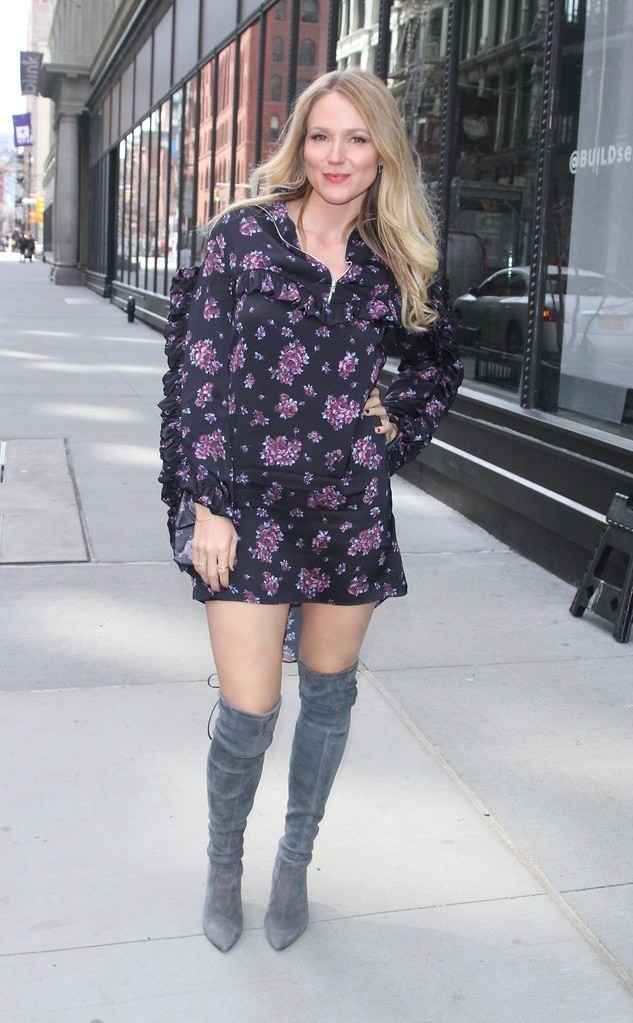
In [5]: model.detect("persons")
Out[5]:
[158,69,463,953]
[28,235,34,262]
[17,233,28,263]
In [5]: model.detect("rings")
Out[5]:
[197,563,207,566]
[217,566,228,569]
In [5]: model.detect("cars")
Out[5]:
[451,266,633,361]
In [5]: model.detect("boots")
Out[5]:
[264,656,360,950]
[202,673,283,953]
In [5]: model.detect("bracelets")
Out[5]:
[196,515,217,521]
